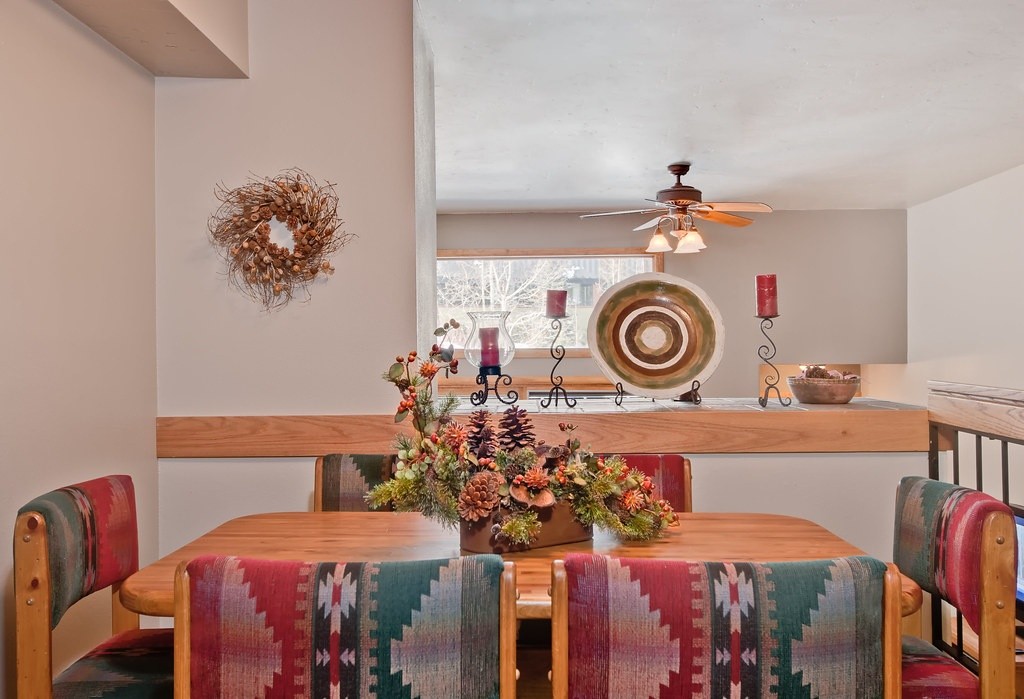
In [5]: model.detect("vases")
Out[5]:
[460,500,594,552]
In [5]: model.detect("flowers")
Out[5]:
[363,350,681,548]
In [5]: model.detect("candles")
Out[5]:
[546,288,567,316]
[478,327,499,366]
[756,273,778,317]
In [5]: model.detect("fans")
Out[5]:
[580,164,772,230]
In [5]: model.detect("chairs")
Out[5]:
[174,553,519,699]
[315,452,400,513]
[549,557,902,699]
[13,473,177,699]
[883,473,1017,699]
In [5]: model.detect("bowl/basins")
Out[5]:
[785,376,860,403]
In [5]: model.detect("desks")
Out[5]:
[120,510,925,623]
[598,454,694,513]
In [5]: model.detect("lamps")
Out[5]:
[645,211,707,254]
